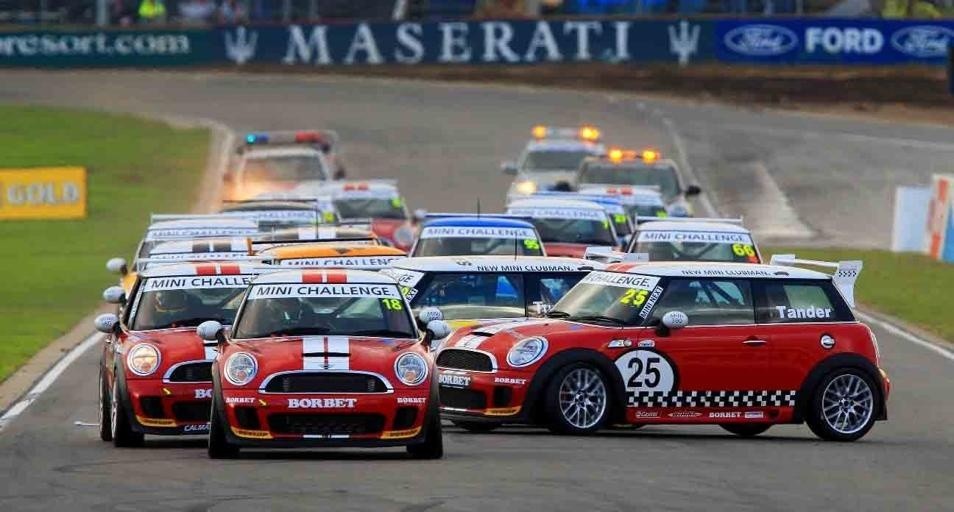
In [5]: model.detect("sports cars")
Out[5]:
[433,254,890,443]
[92,260,282,448]
[195,269,451,459]
